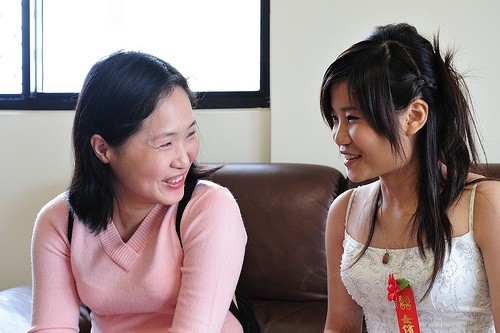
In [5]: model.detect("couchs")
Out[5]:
[80,163,500,333]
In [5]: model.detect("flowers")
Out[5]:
[385,273,410,302]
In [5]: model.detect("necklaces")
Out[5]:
[375,170,456,263]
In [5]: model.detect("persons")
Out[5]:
[321,23,499,333]
[28,51,250,332]
